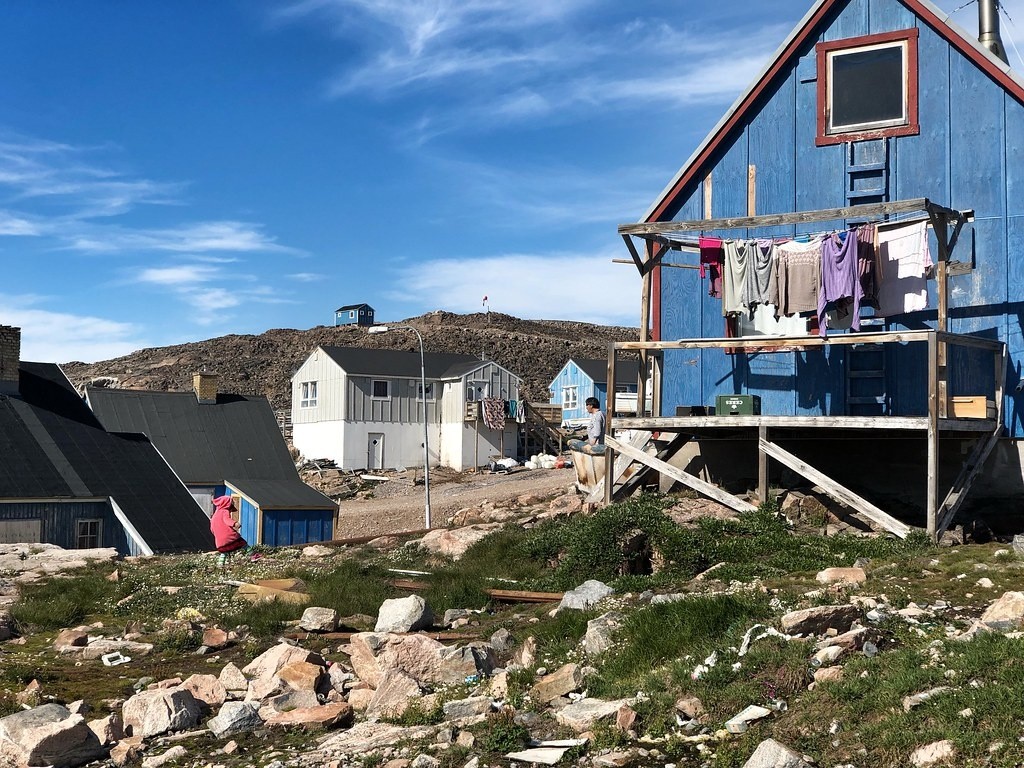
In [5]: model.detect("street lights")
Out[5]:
[368,326,431,531]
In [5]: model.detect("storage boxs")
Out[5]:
[947,396,996,419]
[676,406,715,416]
[715,394,761,416]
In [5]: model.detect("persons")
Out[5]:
[210,495,248,553]
[585,397,606,444]
